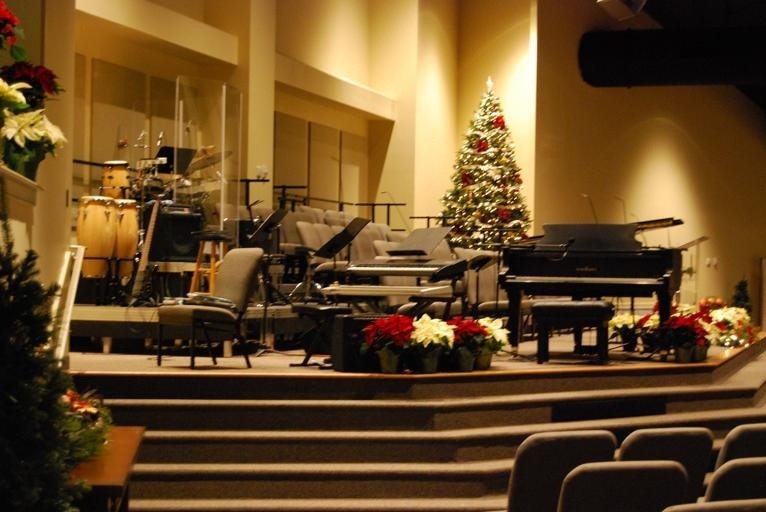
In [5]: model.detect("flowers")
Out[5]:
[361,315,511,353]
[0,2,67,184]
[610,298,763,364]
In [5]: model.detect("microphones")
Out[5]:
[156,132,166,147]
[561,237,576,250]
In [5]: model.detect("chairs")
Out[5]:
[218,204,596,342]
[506,422,765,512]
[154,246,263,370]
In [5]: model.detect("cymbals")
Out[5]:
[190,151,231,170]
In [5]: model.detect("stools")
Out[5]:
[530,301,617,365]
[289,302,352,369]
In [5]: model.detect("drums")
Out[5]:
[101,160,130,199]
[76,196,116,278]
[114,199,140,282]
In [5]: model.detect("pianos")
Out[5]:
[496,219,687,362]
[335,256,468,279]
[319,285,455,301]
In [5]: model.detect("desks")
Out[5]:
[63,424,147,511]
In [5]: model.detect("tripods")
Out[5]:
[254,245,286,356]
[608,297,650,352]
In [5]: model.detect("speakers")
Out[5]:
[332,312,388,371]
[140,207,202,262]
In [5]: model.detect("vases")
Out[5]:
[377,345,494,374]
